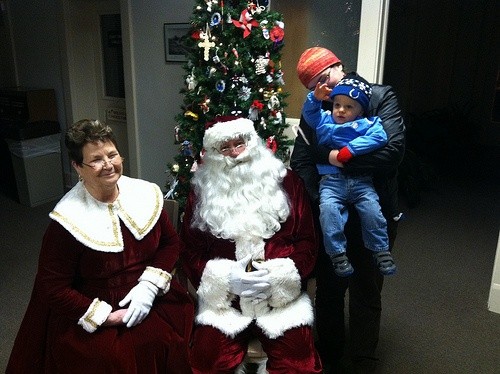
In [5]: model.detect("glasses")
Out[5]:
[213,139,248,155]
[82,152,125,170]
[310,67,331,91]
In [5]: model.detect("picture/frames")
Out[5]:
[163,22,193,63]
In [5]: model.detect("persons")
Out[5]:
[179,113,324,374]
[300,71,398,276]
[31,116,196,374]
[285,48,407,374]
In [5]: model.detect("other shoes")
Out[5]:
[330,253,354,276]
[372,251,397,275]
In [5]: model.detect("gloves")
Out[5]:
[241,261,272,304]
[336,146,353,162]
[118,281,159,328]
[228,254,270,300]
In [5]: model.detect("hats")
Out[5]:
[330,71,374,113]
[297,46,341,89]
[203,115,254,150]
